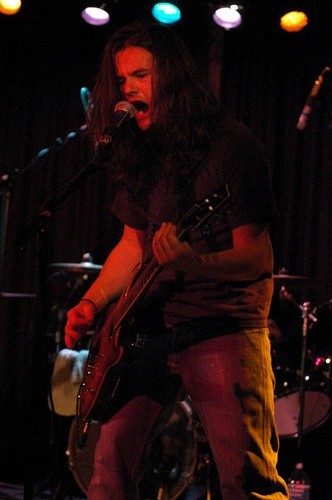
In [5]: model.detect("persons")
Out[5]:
[63,21,292,500]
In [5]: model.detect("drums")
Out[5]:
[273,337,332,437]
[67,391,199,500]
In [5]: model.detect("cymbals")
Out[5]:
[273,273,312,284]
[50,258,104,274]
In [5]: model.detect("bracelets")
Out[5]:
[80,298,98,315]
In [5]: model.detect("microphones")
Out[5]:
[95,101,135,155]
[297,67,329,130]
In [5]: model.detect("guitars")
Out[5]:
[76,181,240,425]
